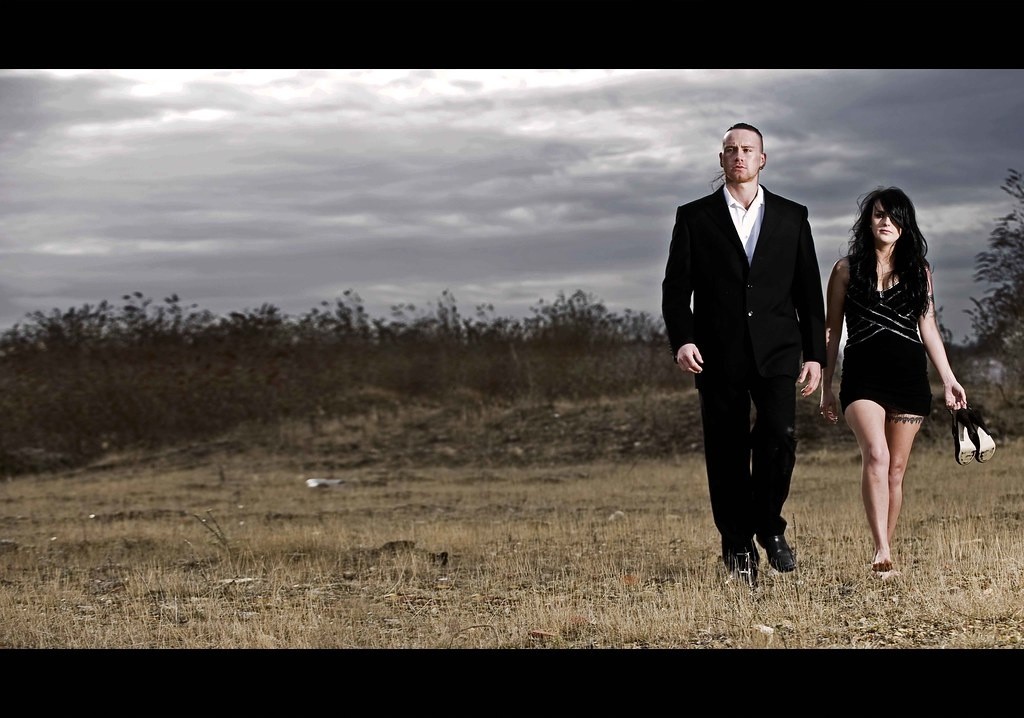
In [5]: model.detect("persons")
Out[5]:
[659,122,826,584]
[816,185,970,578]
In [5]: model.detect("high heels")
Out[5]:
[952,409,977,465]
[968,409,996,464]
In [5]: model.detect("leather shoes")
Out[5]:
[755,534,796,572]
[727,556,756,588]
